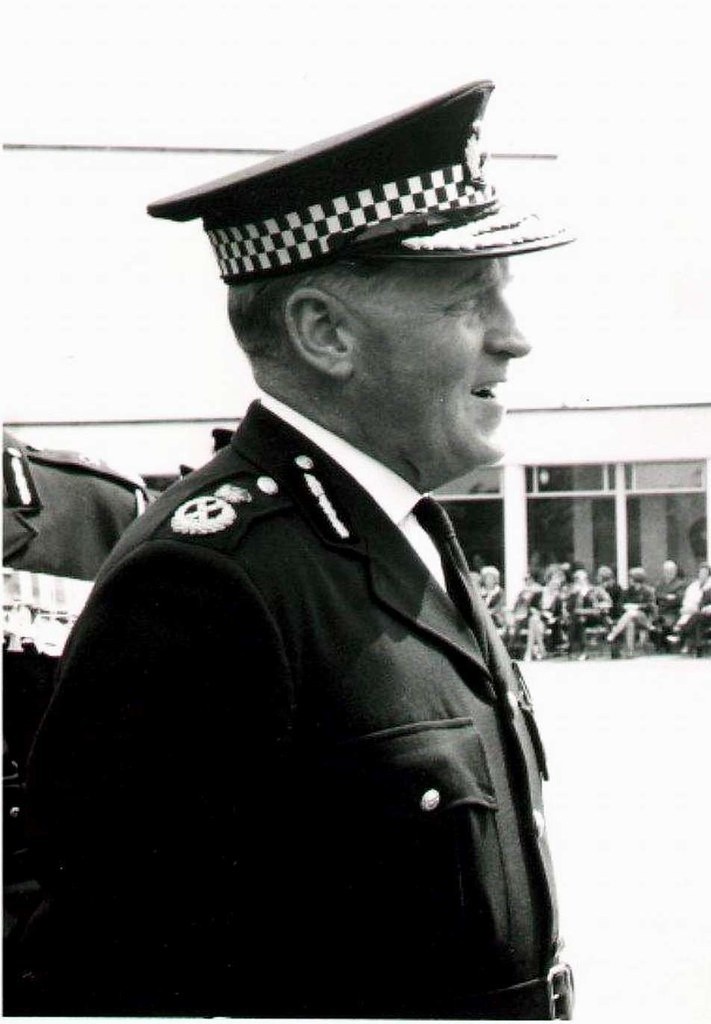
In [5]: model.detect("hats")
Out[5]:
[147,79,578,288]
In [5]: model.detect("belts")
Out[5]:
[460,962,577,1021]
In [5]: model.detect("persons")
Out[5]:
[469,559,711,662]
[2,430,162,1018]
[25,77,576,1021]
